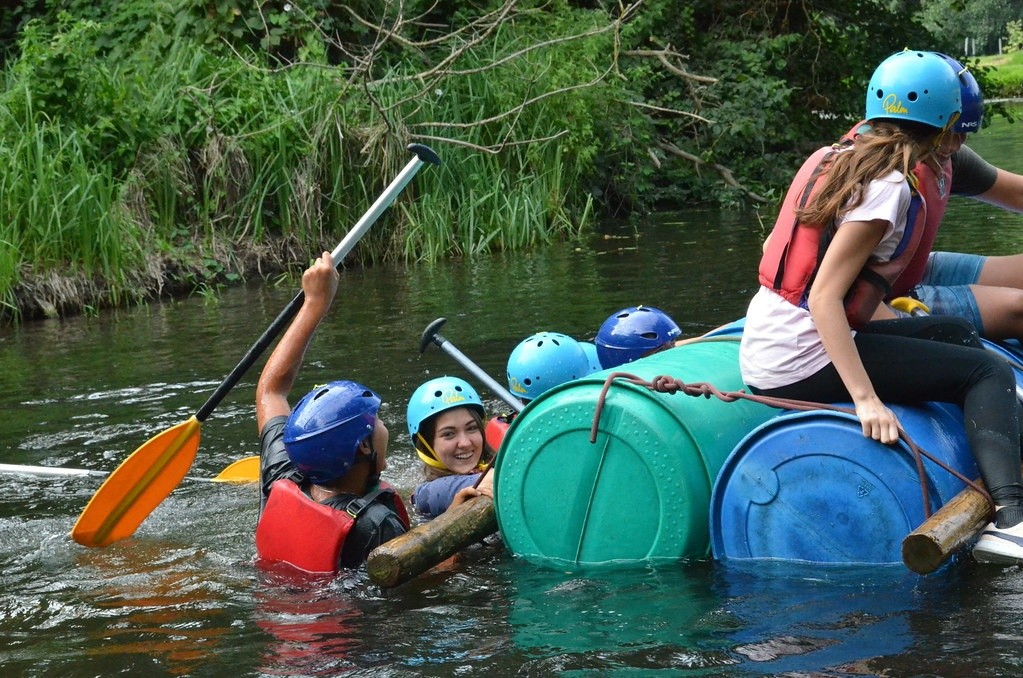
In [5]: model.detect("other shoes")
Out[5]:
[973,521,1023,564]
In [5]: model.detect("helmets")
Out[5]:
[282,380,381,484]
[864,46,962,129]
[594,305,681,369]
[577,341,603,374]
[407,375,487,440]
[936,50,981,132]
[506,331,589,402]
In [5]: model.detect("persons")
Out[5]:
[739,51,1023,565]
[253,251,682,567]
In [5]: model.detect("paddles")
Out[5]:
[214,453,261,485]
[70,139,447,552]
[890,292,1023,407]
[416,312,531,415]
